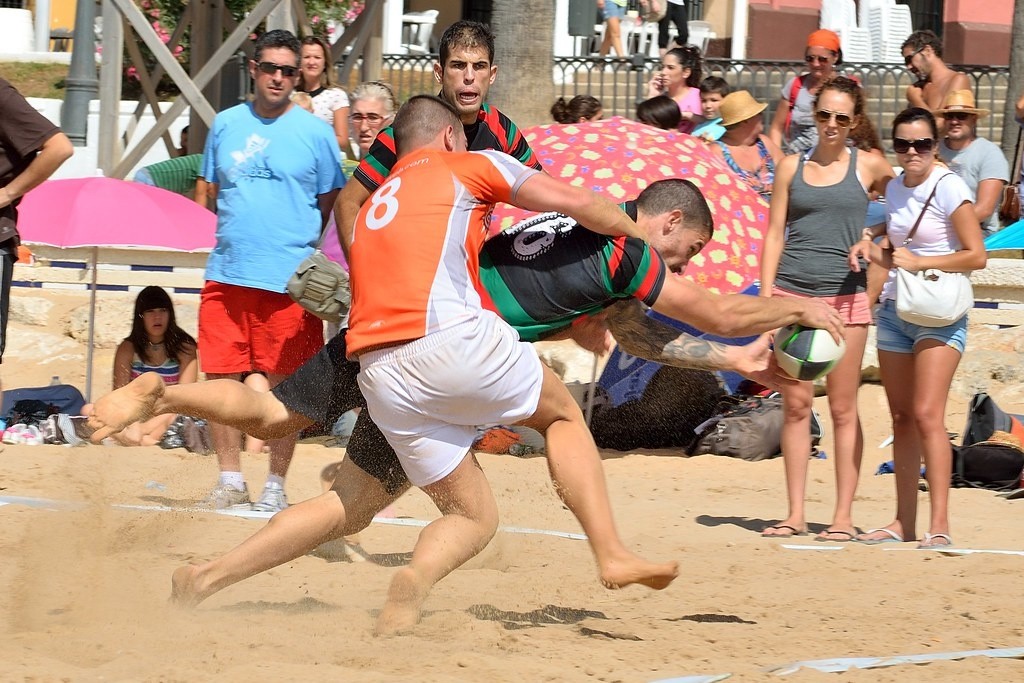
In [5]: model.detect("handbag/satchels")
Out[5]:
[895,266,974,327]
[963,392,1024,489]
[285,250,351,323]
[647,0,668,23]
[998,184,1021,226]
[39,414,111,447]
[179,417,216,456]
[918,442,1024,495]
[5,399,61,429]
[685,379,825,461]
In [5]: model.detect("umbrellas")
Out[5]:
[15,175,219,404]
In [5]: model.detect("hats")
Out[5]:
[932,89,992,119]
[718,90,768,126]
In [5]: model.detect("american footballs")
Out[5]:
[772,323,846,382]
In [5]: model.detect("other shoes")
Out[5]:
[2,424,43,445]
[595,63,604,71]
[616,63,629,71]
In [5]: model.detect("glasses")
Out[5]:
[905,46,924,65]
[347,112,392,126]
[255,60,301,76]
[943,113,976,120]
[893,137,937,154]
[813,107,854,128]
[805,54,836,64]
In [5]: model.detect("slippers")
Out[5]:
[814,528,861,541]
[916,532,954,549]
[761,525,808,538]
[850,528,904,545]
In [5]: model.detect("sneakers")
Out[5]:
[250,487,289,511]
[195,481,253,509]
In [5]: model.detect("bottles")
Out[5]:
[50,375,60,385]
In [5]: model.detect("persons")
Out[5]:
[597,0,628,61]
[848,106,988,550]
[113,285,198,447]
[651,0,689,59]
[240,21,1024,454]
[0,76,74,366]
[79,179,847,610]
[758,76,897,541]
[345,94,681,643]
[197,28,349,512]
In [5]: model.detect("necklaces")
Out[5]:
[149,341,164,350]
